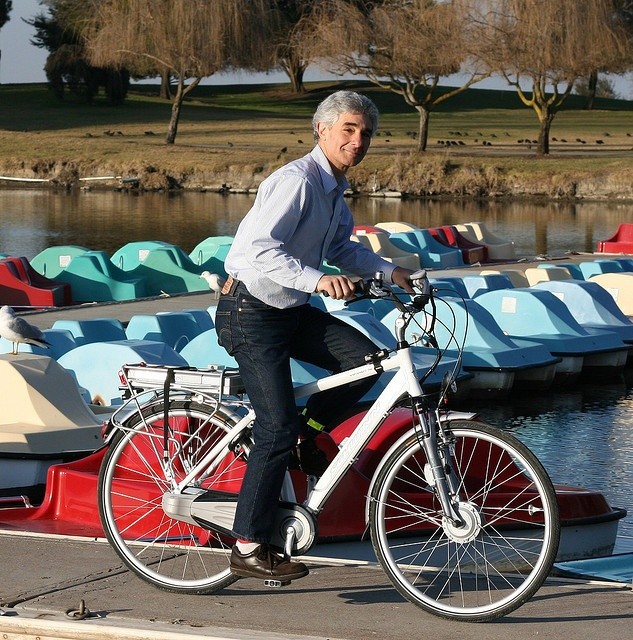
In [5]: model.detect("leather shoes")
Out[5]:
[230,543,309,581]
[290,438,330,476]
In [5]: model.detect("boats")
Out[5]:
[583,258,633,265]
[0,350,141,493]
[0,244,96,308]
[463,272,632,369]
[537,265,633,280]
[310,280,565,397]
[96,271,227,302]
[96,236,233,268]
[0,309,189,404]
[127,305,477,414]
[322,218,516,275]
[1,400,628,578]
[390,275,633,384]
[480,264,633,330]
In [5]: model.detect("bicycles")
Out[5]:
[95,269,561,624]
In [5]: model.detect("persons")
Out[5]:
[215,91,428,582]
